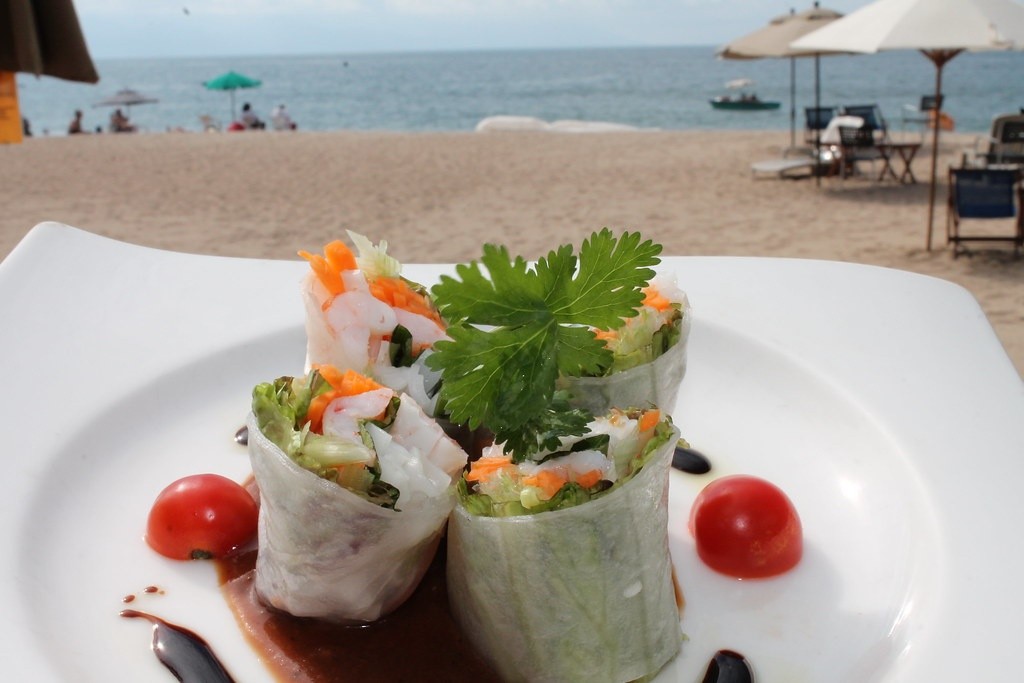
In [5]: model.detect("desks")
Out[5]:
[873,140,921,184]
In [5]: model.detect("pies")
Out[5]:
[242,228,694,683]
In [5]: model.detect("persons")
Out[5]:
[721,95,730,102]
[96,126,104,134]
[241,103,265,130]
[270,104,293,130]
[22,117,33,137]
[68,110,82,134]
[111,109,136,132]
[749,95,759,104]
[738,94,749,103]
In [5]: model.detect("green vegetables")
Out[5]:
[430,225,662,464]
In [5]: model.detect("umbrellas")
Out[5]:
[789,0,1024,251]
[202,70,264,123]
[715,0,862,190]
[92,85,158,124]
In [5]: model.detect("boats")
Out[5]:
[708,81,780,110]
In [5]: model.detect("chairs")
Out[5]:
[945,115,1024,261]
[751,103,888,181]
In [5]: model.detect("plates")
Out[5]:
[0,219,1024,683]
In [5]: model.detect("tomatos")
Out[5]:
[144,473,258,560]
[687,474,803,578]
